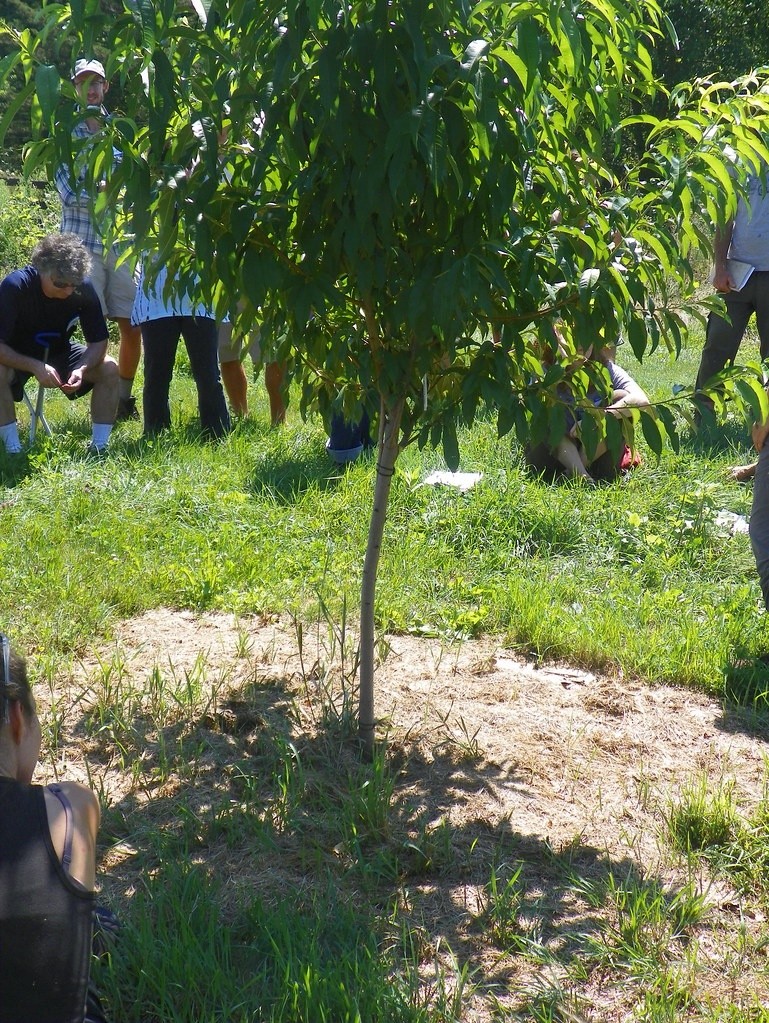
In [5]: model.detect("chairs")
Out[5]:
[20,330,63,449]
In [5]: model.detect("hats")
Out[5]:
[70,59,107,80]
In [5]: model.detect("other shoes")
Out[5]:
[620,444,642,467]
[118,396,138,420]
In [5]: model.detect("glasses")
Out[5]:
[50,277,80,289]
[74,80,103,87]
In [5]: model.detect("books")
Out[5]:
[706,259,755,291]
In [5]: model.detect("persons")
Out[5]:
[50,59,142,421]
[680,145,769,420]
[130,222,232,448]
[218,312,290,432]
[0,232,121,456]
[522,311,649,489]
[748,383,769,613]
[326,405,375,465]
[0,634,101,1023]
